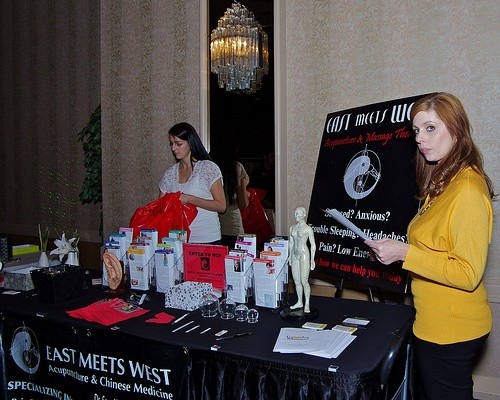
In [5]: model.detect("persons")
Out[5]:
[157,121,226,245]
[363,92,500,400]
[209,135,251,248]
[288,207,316,313]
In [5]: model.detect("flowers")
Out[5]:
[49,229,80,262]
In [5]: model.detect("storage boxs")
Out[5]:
[2,260,86,304]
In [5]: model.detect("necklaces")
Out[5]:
[419,197,437,215]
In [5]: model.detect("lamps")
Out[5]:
[210,0,269,94]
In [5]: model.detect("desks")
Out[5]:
[0,286,418,400]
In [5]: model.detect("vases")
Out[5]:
[65,252,79,266]
[39,252,50,267]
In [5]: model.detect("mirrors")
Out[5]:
[200,0,287,258]
